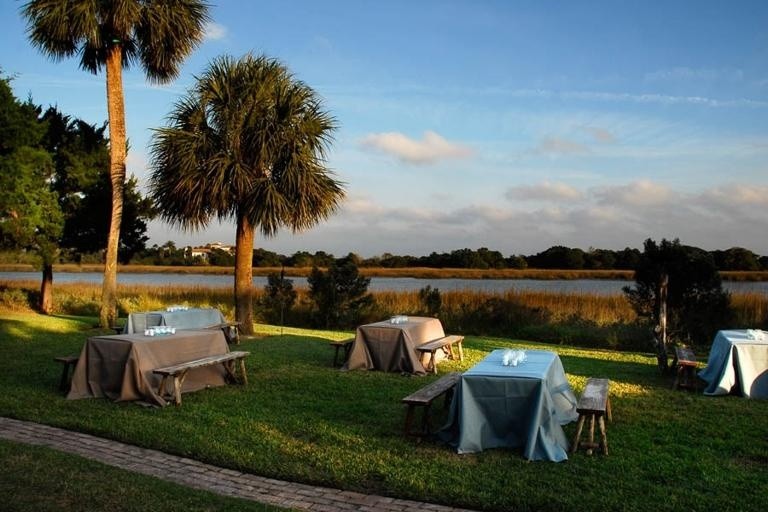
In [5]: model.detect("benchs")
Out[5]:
[668,344,698,395]
[110,326,123,334]
[568,377,613,457]
[52,353,78,393]
[152,352,255,408]
[416,334,464,374]
[209,320,243,345]
[327,336,356,367]
[399,372,460,439]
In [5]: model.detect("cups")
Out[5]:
[390,314,408,325]
[143,326,176,337]
[502,349,526,366]
[746,328,765,340]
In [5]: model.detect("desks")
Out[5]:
[451,348,562,464]
[355,313,440,377]
[123,305,227,334]
[78,327,237,402]
[711,329,767,403]
